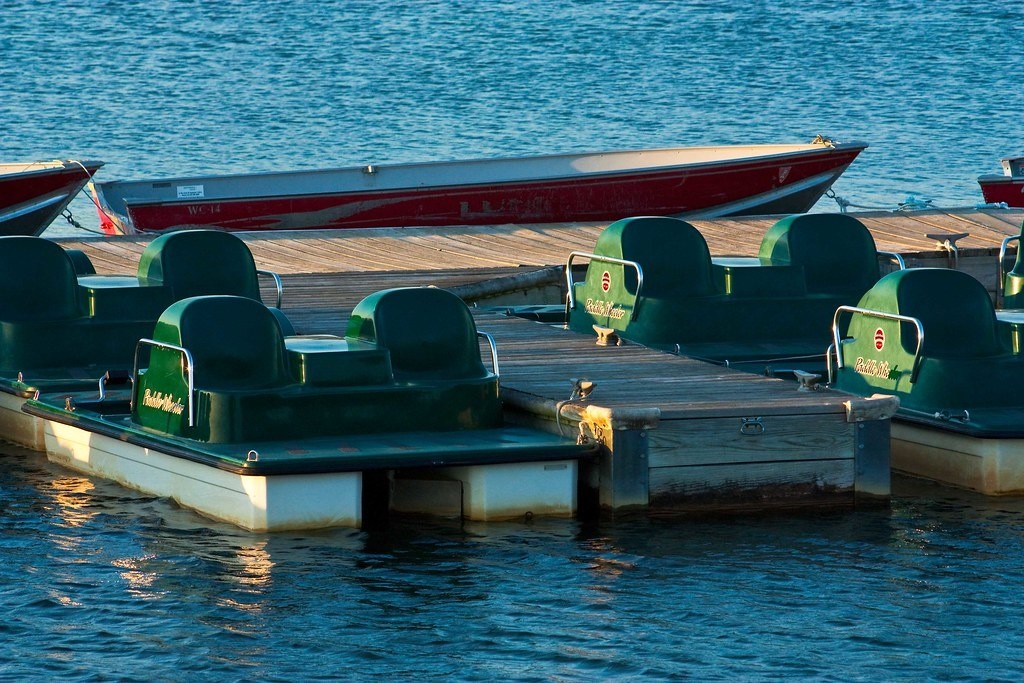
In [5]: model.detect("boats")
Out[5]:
[83,131,871,241]
[507,213,1024,504]
[0,161,107,237]
[0,223,601,540]
[978,156,1024,209]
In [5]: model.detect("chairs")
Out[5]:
[139,220,293,334]
[145,289,293,439]
[0,234,88,371]
[853,258,1007,419]
[998,217,1024,310]
[586,216,715,354]
[343,281,507,427]
[760,205,900,351]
